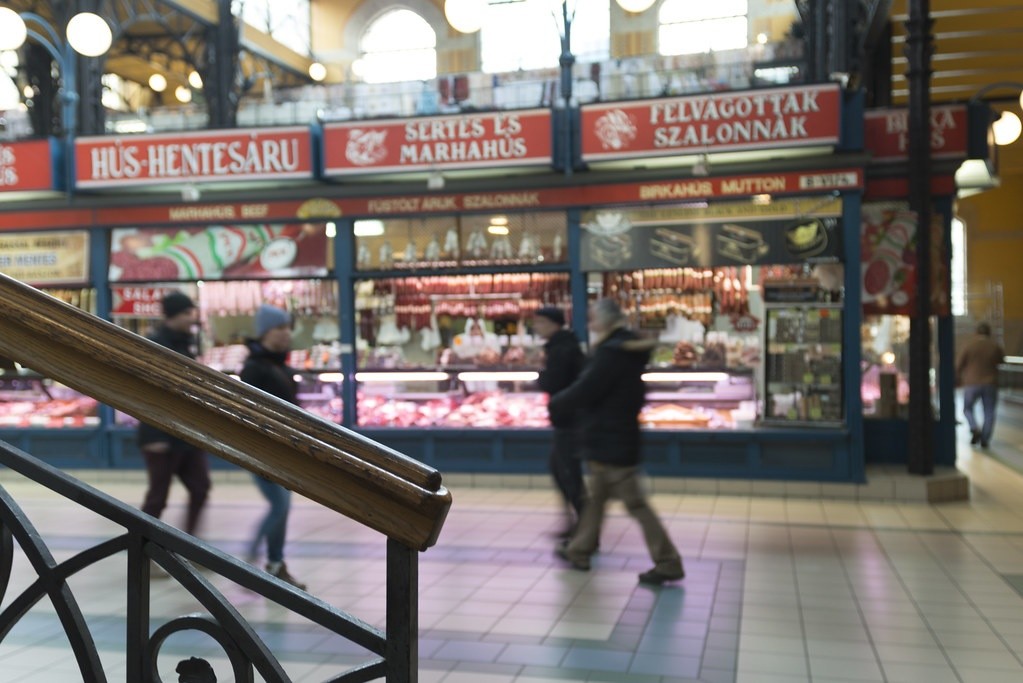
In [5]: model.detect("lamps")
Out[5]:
[974,82,1023,146]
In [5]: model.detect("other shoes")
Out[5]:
[556,544,589,571]
[150,559,169,578]
[557,526,577,538]
[265,561,307,590]
[637,568,685,586]
[971,431,981,444]
[980,441,987,447]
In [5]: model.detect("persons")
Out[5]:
[520,305,590,538]
[236,304,305,590]
[134,290,213,579]
[545,298,686,585]
[956,323,1005,447]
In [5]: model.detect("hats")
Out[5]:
[538,307,567,327]
[162,294,195,320]
[253,304,291,341]
